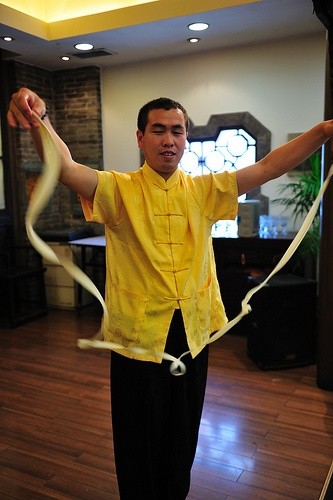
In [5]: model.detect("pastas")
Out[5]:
[26,111,333,377]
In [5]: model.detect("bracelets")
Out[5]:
[41,104,49,120]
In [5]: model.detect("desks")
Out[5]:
[67,236,107,316]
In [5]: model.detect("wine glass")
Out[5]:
[259,215,289,235]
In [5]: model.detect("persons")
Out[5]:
[8,87,333,500]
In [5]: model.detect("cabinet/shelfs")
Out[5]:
[246,274,317,370]
[212,231,317,334]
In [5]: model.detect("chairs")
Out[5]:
[0,224,48,330]
